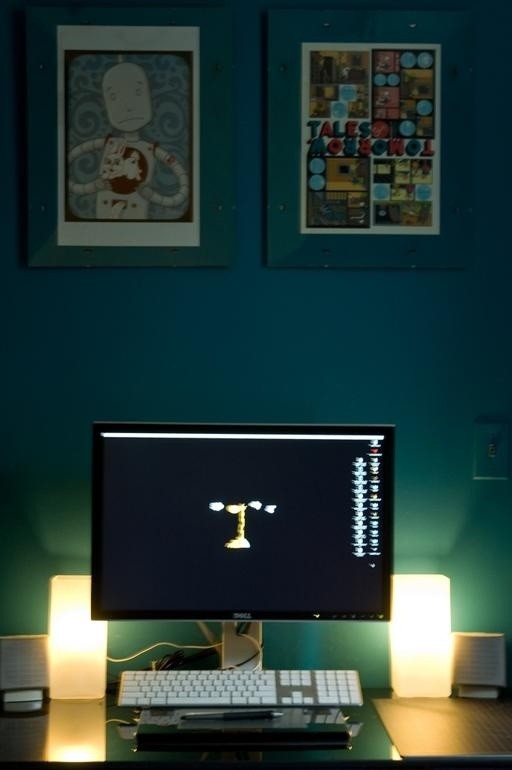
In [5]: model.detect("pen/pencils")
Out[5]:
[180,711,283,721]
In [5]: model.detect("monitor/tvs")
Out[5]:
[91,420,396,670]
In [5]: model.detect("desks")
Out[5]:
[1,689,512,770]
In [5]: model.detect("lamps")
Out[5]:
[47,575,108,700]
[45,696,106,764]
[389,573,454,697]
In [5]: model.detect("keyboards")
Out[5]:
[117,669,363,709]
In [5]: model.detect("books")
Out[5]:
[133,707,351,752]
[370,692,512,760]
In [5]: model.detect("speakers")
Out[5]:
[0,636,49,702]
[451,632,508,698]
